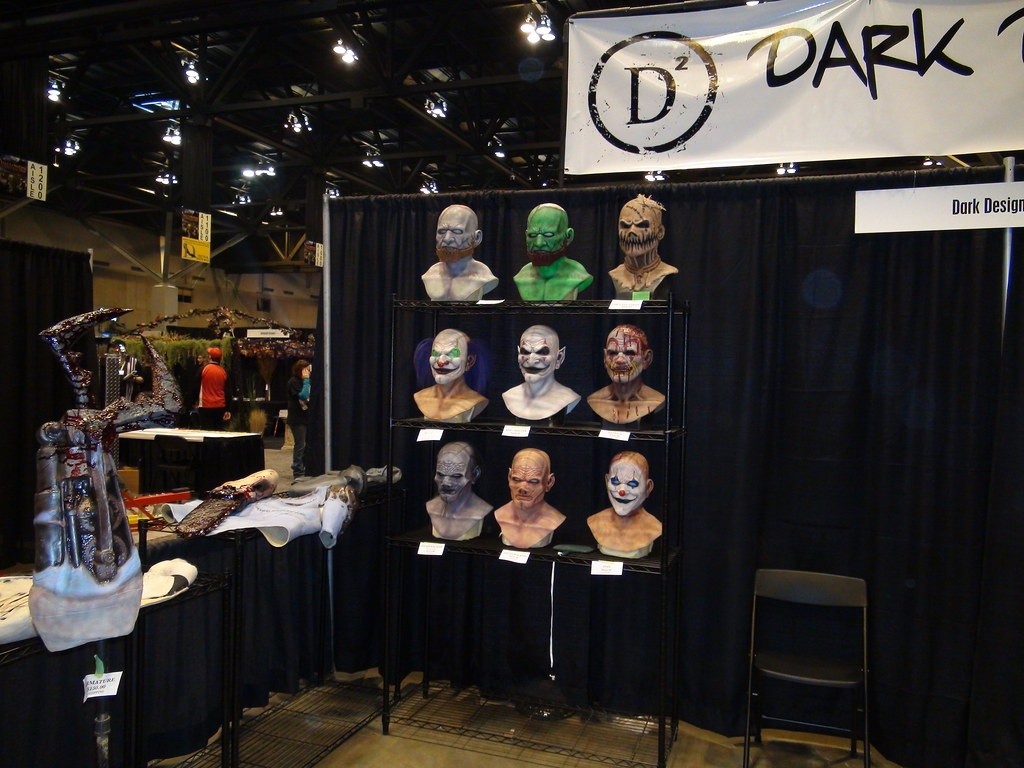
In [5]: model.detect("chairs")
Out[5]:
[742,567,873,768]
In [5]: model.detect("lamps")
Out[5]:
[42,0,799,202]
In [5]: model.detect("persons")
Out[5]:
[198,346,233,430]
[112,341,145,400]
[411,193,682,559]
[286,359,314,481]
[194,354,206,382]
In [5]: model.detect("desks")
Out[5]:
[120,428,267,499]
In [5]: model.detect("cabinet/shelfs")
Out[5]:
[380,287,697,768]
[131,475,406,768]
[0,568,232,768]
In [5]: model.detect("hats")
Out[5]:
[207,347,222,360]
